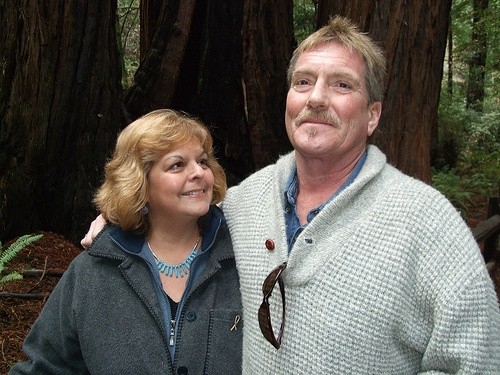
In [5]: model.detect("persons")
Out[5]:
[82,16,500,375]
[6,106,245,375]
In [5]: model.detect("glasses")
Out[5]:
[257,261,288,349]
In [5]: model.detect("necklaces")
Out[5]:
[142,207,202,274]
